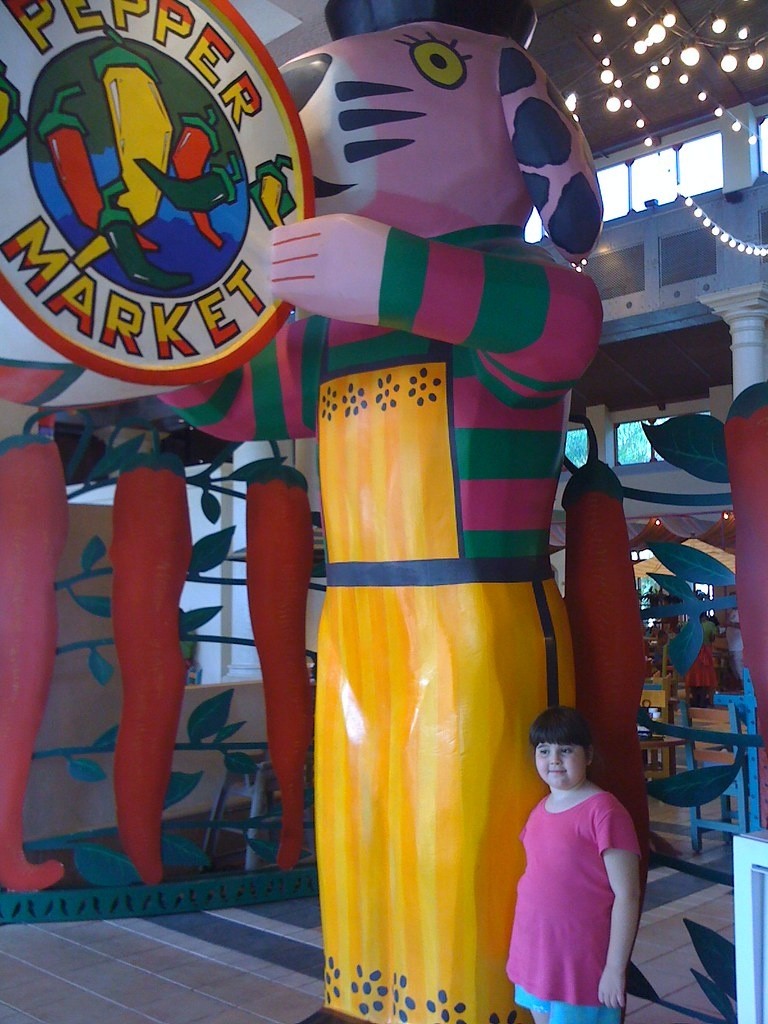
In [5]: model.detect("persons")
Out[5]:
[653,591,743,706]
[505,706,641,1024]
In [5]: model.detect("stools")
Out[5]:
[200,752,320,873]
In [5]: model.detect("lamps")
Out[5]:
[644,199,658,207]
[724,512,731,520]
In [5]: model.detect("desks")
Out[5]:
[638,736,688,780]
